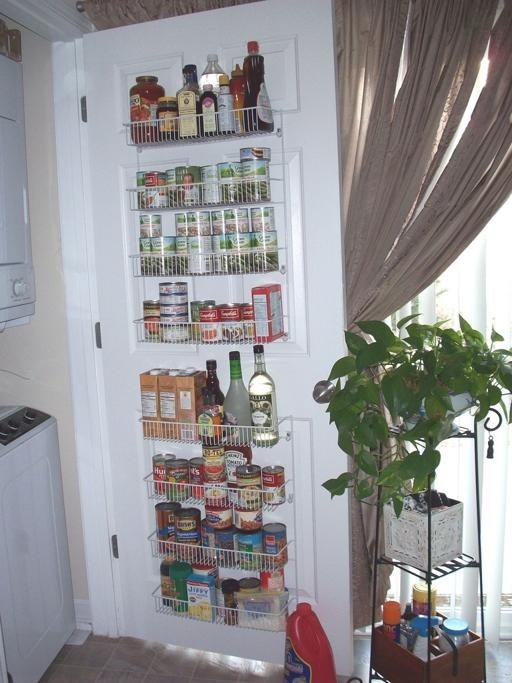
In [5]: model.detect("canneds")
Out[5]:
[156,97,177,140]
[153,445,288,570]
[216,577,261,626]
[137,147,279,276]
[142,283,255,343]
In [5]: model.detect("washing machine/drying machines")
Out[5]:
[0,22,39,331]
[0,407,79,683]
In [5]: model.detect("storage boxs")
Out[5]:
[368,612,486,683]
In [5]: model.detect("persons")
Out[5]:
[178,174,198,206]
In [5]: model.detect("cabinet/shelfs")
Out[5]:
[367,390,503,683]
[121,105,292,635]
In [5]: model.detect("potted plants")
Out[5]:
[313,312,511,572]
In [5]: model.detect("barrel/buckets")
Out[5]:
[284,601,337,682]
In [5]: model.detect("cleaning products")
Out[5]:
[410,615,444,664]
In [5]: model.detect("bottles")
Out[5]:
[381,580,470,660]
[175,40,275,141]
[248,344,281,448]
[205,359,226,436]
[222,350,253,444]
[197,385,221,446]
[159,555,180,608]
